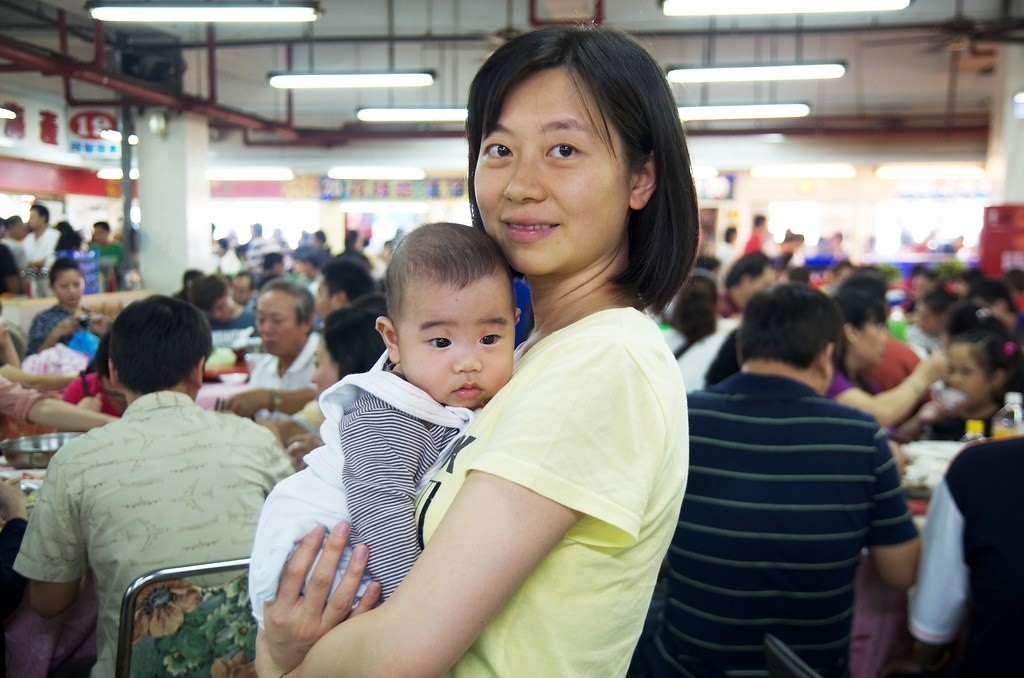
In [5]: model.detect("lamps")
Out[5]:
[665,14,849,84]
[266,0,436,90]
[82,0,327,23]
[355,0,469,122]
[678,82,805,124]
[657,0,915,16]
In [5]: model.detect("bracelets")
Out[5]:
[272,388,281,411]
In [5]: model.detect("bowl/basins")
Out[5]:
[219,373,248,384]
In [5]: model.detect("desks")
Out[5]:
[898,440,969,531]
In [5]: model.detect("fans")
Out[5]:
[857,0,1024,56]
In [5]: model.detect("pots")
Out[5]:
[0,432,84,469]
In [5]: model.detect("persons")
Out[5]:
[248,221,522,611]
[254,20,698,678]
[0,203,396,677]
[626,215,1024,678]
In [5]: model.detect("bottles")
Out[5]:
[992,392,1024,440]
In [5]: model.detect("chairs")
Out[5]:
[114,559,257,678]
